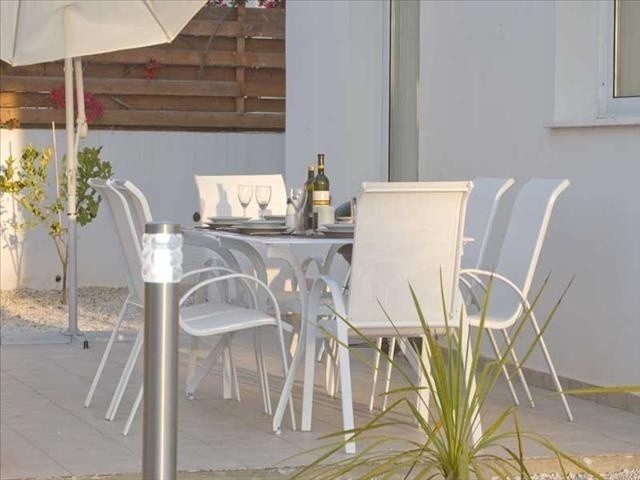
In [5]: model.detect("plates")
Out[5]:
[205,217,289,235]
[319,222,355,238]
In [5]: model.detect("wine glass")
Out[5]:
[289,186,308,235]
[255,184,272,221]
[237,183,254,220]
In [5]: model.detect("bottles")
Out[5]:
[312,153,330,232]
[305,166,317,231]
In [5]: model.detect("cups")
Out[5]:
[316,206,335,233]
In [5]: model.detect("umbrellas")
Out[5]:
[0,0,207,338]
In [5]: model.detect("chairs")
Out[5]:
[332,175,520,413]
[111,179,297,435]
[189,173,328,414]
[300,180,484,455]
[77,172,270,422]
[381,178,577,427]
